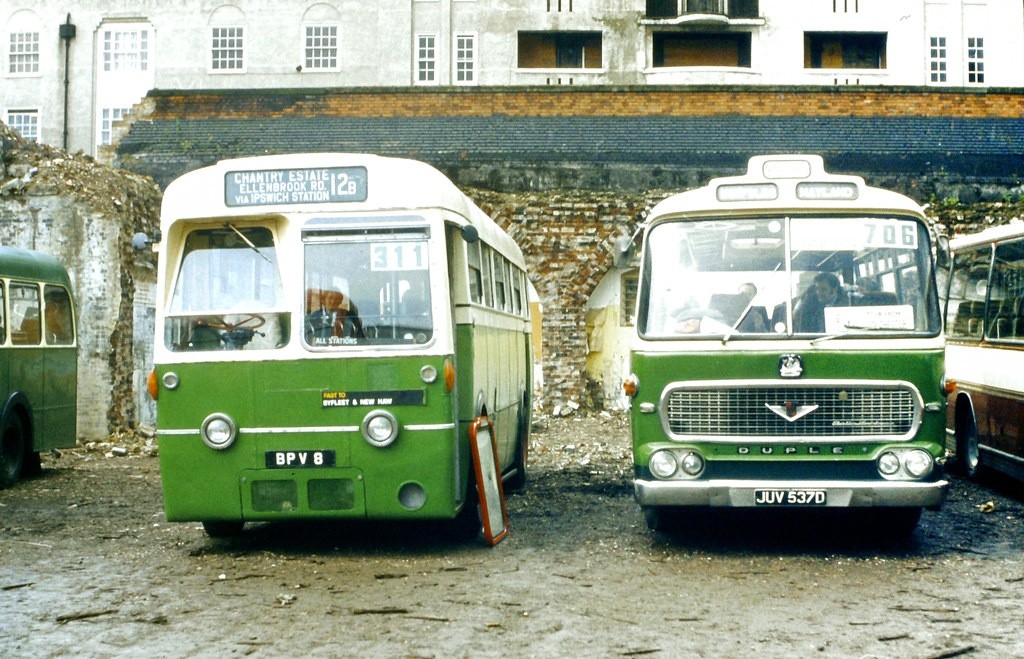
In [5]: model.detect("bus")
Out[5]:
[0,246,77,490]
[129,151,534,544]
[613,156,956,541]
[931,213,1023,486]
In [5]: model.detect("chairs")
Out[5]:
[217,300,282,351]
[952,295,1024,339]
[306,319,378,339]
[711,292,897,334]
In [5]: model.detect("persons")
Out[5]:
[738,283,769,330]
[857,274,879,297]
[808,272,851,328]
[309,287,365,338]
